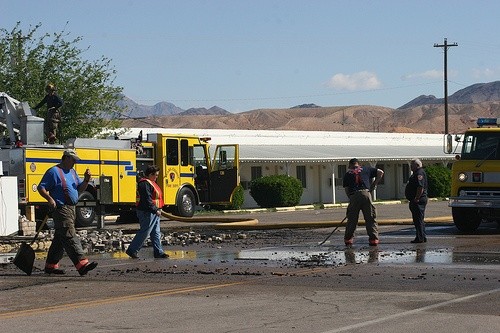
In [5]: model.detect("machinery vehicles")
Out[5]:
[443,117,500,231]
[0,91,241,227]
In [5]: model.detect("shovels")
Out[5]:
[319,171,382,245]
[13,206,57,275]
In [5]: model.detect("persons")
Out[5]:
[125,164,170,259]
[30,84,63,144]
[404,159,428,243]
[343,158,384,245]
[37,149,98,276]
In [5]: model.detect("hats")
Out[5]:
[63,149,81,161]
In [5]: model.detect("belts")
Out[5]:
[350,189,369,194]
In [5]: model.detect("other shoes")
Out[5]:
[44,267,66,274]
[78,261,98,276]
[125,250,140,259]
[344,236,353,244]
[368,239,379,246]
[411,238,427,243]
[154,253,170,258]
